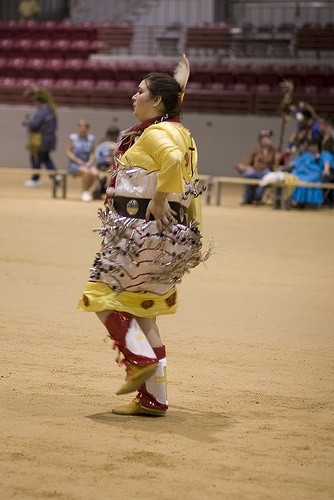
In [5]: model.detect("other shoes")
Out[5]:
[116,363,158,396]
[25,180,40,186]
[112,398,165,417]
[81,192,92,201]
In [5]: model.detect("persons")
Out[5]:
[77,72,201,418]
[236,102,334,210]
[66,116,120,203]
[21,88,64,187]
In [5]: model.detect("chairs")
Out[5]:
[0,19,334,118]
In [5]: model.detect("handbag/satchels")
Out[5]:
[25,132,41,150]
